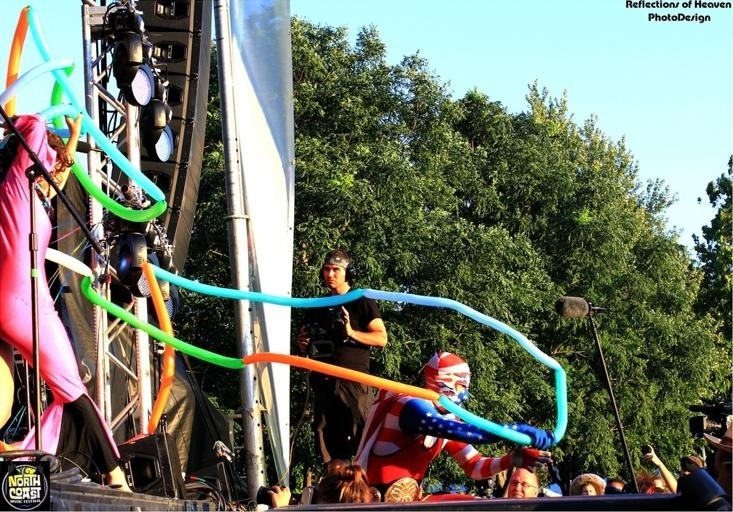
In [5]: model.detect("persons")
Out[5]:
[0,111,136,494]
[352,351,556,504]
[313,413,332,462]
[295,246,389,457]
[500,422,732,511]
[267,414,380,509]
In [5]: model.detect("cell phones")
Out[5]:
[641,445,650,455]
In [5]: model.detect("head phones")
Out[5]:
[317,250,359,282]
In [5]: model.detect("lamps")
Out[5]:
[109,5,177,168]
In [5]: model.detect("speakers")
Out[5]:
[118,430,186,501]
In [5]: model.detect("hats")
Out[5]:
[703,415,733,453]
[569,473,606,496]
[679,456,704,469]
